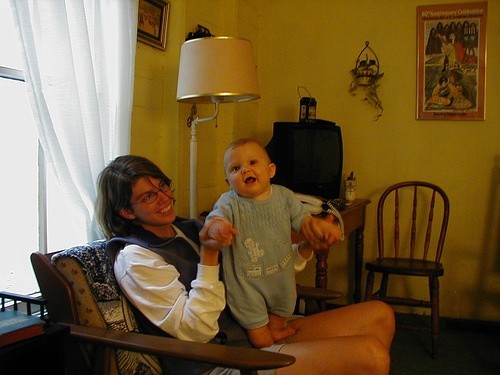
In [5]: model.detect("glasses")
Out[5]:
[127,180,172,205]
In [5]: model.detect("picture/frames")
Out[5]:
[415,0,490,121]
[137,0,171,52]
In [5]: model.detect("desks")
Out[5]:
[200,199,372,312]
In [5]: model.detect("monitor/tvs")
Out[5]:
[264,121,343,202]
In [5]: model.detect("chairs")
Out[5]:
[30,217,344,375]
[364,180,450,359]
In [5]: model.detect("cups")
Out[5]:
[344,180,357,201]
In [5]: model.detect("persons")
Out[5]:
[92,155,396,375]
[205,139,341,352]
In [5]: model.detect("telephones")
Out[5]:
[294,192,324,215]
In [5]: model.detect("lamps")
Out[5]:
[176,36,261,219]
